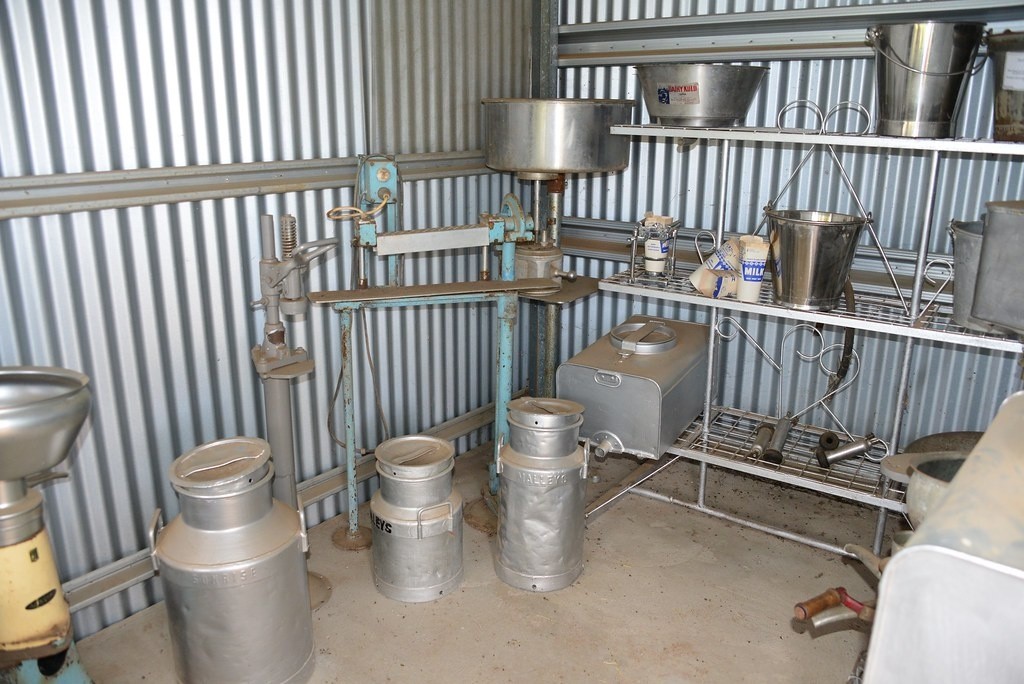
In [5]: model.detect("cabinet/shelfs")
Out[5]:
[597,123,1024,564]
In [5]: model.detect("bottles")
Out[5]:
[645,219,669,277]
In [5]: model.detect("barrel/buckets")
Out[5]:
[979,28,1024,143]
[969,199,1024,333]
[864,20,991,137]
[763,201,874,311]
[946,220,1016,336]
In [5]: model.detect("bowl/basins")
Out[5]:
[632,64,770,126]
